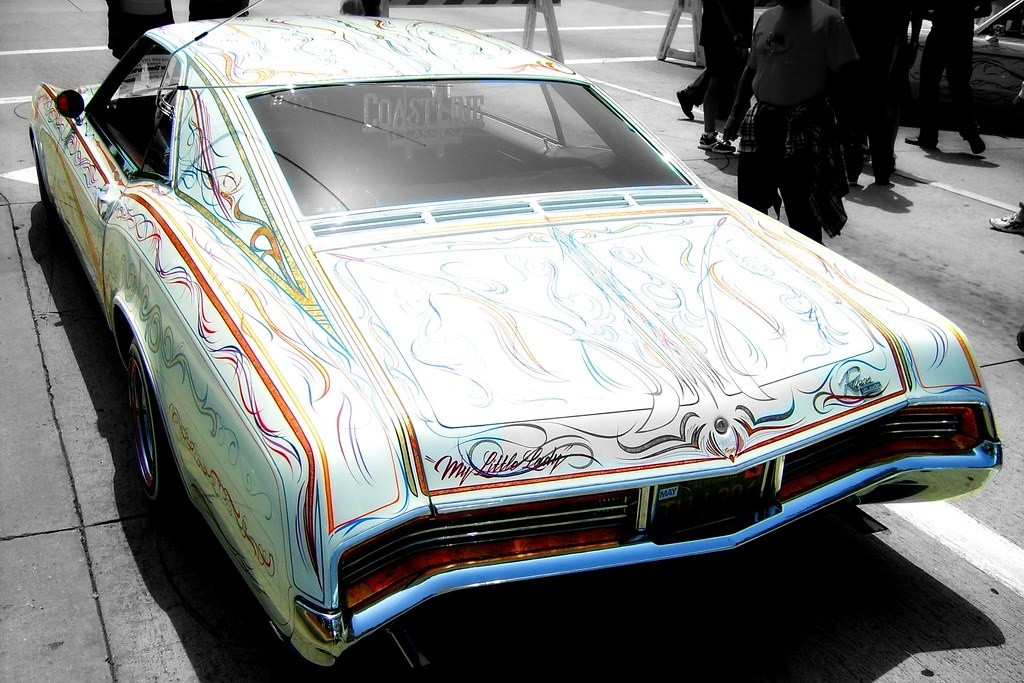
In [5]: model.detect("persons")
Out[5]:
[677,0,993,189]
[989,81,1024,234]
[722,0,863,247]
[106,0,175,97]
[189,0,249,22]
[340,0,381,17]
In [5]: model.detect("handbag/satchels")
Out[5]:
[735,34,750,65]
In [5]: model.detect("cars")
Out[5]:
[908,1,1024,108]
[31,10,1006,678]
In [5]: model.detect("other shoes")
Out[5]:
[905,135,936,149]
[964,127,986,154]
[677,87,695,121]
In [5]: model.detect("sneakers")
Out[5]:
[990,202,1024,234]
[699,132,736,152]
